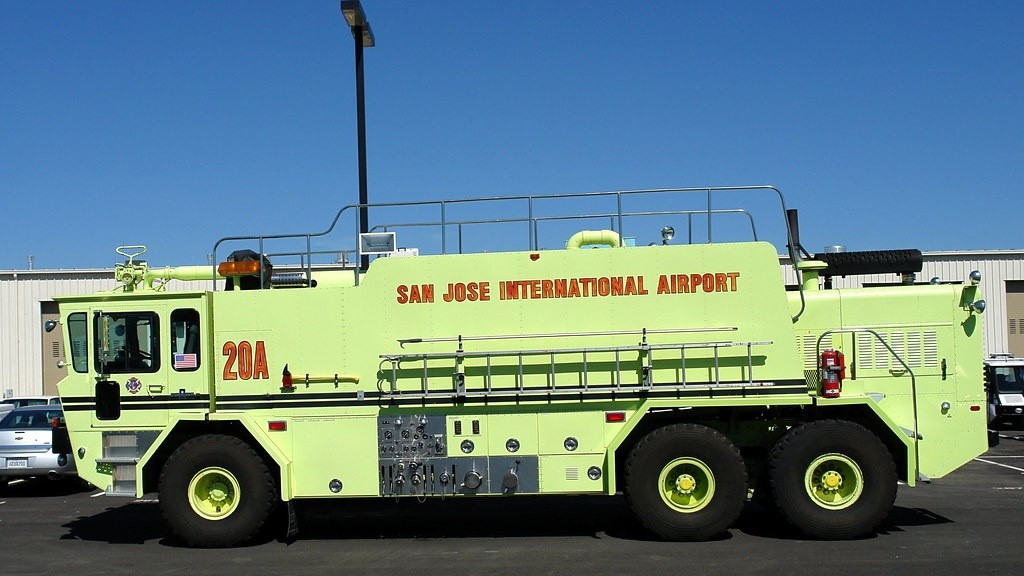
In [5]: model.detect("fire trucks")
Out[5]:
[45,186,988,549]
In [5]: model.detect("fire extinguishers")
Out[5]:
[822,349,842,398]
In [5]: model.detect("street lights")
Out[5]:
[340,0,374,268]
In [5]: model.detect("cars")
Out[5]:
[0,396,78,491]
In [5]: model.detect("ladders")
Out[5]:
[378,341,775,397]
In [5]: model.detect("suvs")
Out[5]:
[984,352,1024,429]
